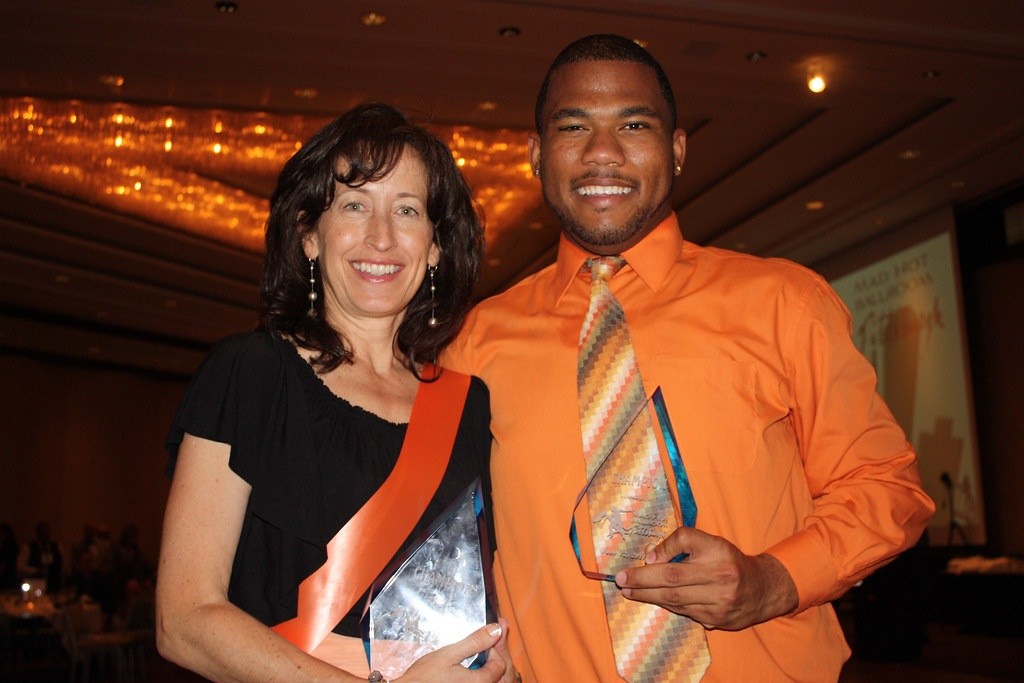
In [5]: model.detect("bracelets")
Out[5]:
[368,669,388,683]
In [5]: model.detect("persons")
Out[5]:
[431,36,937,681]
[0,517,157,652]
[148,98,515,683]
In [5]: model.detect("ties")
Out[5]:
[576,254,713,683]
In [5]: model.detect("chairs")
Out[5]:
[61,602,151,683]
